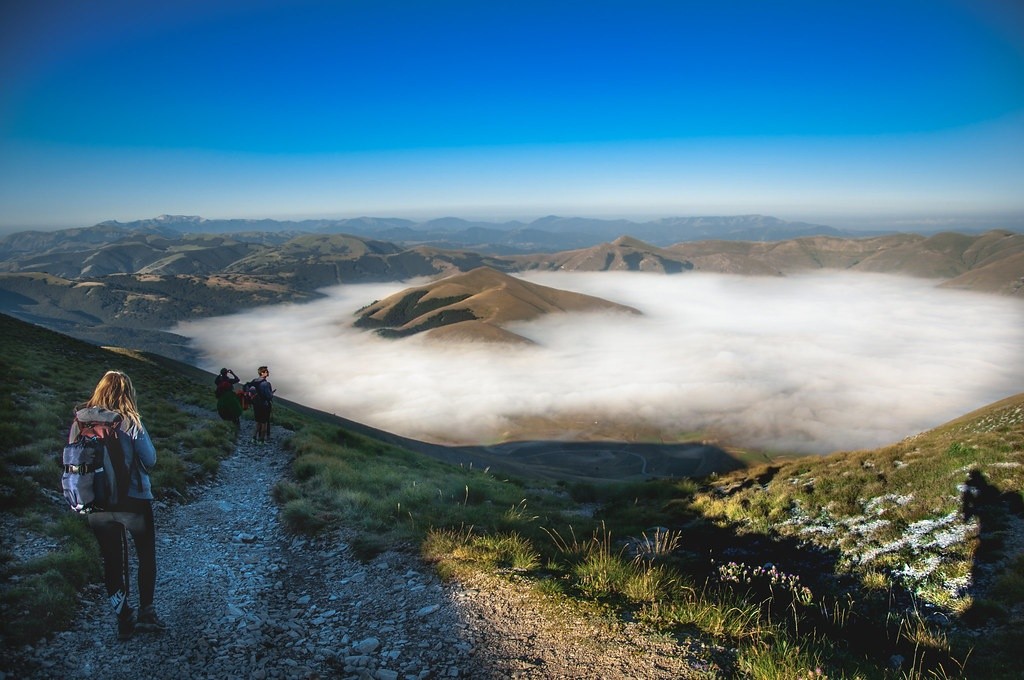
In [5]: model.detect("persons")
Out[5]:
[215,366,273,446]
[69,371,171,640]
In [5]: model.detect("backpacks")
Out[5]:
[243,379,267,404]
[216,379,232,399]
[61,410,128,514]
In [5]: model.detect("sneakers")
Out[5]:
[251,439,266,446]
[117,613,139,639]
[135,606,166,631]
